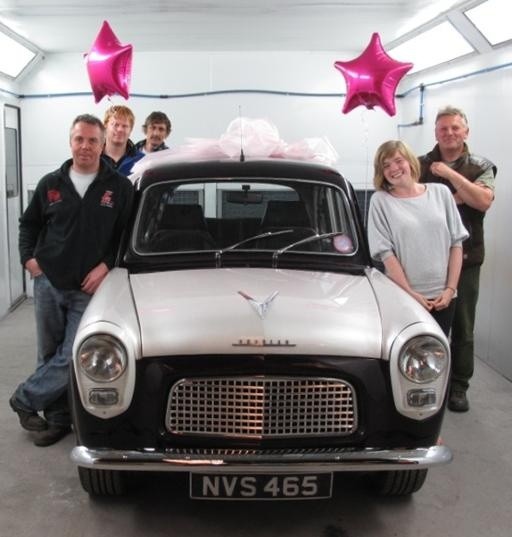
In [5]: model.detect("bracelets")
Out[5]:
[446,286,459,294]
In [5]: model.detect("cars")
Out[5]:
[72,106,451,503]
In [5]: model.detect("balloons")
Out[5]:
[84,21,133,103]
[334,33,413,116]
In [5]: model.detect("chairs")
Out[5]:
[261,200,310,228]
[158,203,208,236]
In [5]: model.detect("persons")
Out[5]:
[101,106,145,178]
[136,112,172,156]
[10,115,134,446]
[416,107,498,412]
[367,141,471,340]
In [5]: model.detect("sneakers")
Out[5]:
[448,391,469,412]
[34,425,72,447]
[9,398,48,430]
